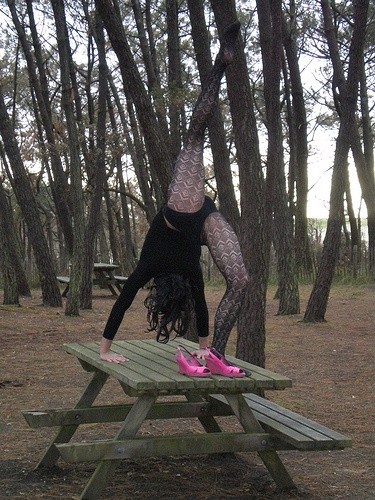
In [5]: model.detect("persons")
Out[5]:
[101,22,252,377]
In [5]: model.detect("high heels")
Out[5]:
[175,344,212,377]
[204,346,246,377]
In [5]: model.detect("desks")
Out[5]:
[35,338,298,500]
[62,263,122,297]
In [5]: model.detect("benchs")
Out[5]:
[105,275,128,281]
[206,392,352,451]
[57,276,71,283]
